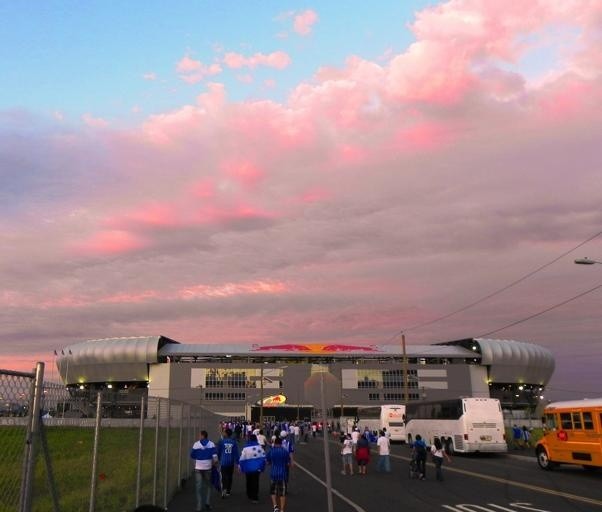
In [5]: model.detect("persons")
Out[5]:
[355,434,369,475]
[339,432,355,476]
[382,427,391,439]
[410,433,427,481]
[430,437,451,482]
[265,437,292,512]
[217,415,371,468]
[236,433,266,504]
[520,425,531,448]
[190,429,220,512]
[511,424,524,451]
[216,428,240,498]
[374,430,391,473]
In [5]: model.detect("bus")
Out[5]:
[331,397,511,457]
[532,397,602,472]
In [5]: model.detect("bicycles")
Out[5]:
[407,449,424,482]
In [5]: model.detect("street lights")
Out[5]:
[572,256,601,266]
[259,360,269,430]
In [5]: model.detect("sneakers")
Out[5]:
[204,503,213,510]
[272,504,280,512]
[222,489,232,498]
